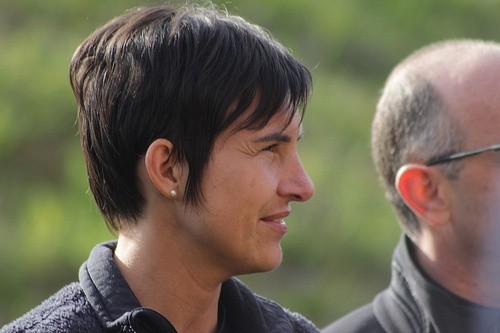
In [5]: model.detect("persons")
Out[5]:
[0,5,322,333]
[323,39,500,333]
